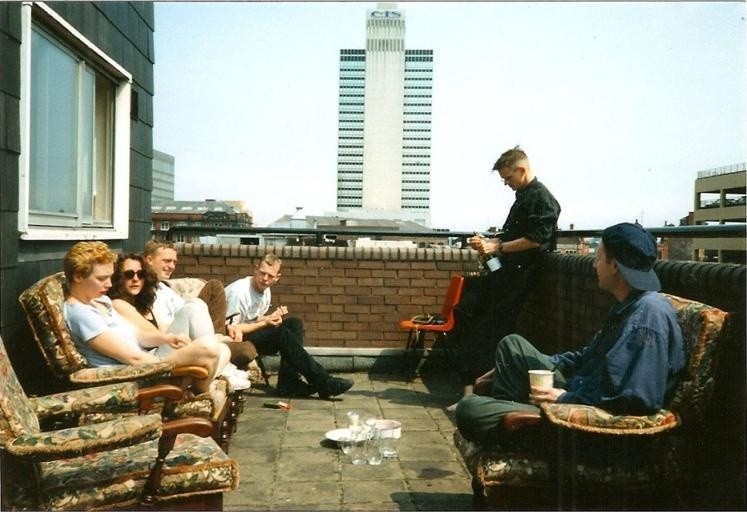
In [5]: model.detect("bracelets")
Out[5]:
[496,238,505,257]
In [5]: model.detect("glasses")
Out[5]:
[120,270,147,279]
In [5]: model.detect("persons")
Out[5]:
[142,237,260,371]
[454,221,688,452]
[63,240,220,405]
[445,143,563,413]
[222,253,357,401]
[108,252,252,392]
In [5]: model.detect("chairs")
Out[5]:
[165,276,270,397]
[400,274,464,384]
[475,293,733,512]
[18,271,237,455]
[0,335,240,511]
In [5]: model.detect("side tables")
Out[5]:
[540,400,679,512]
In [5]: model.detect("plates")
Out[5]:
[325,427,374,444]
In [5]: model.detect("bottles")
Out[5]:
[471,230,503,273]
[290,207,307,233]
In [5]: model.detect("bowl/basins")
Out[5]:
[366,420,402,438]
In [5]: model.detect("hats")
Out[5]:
[602,222,662,291]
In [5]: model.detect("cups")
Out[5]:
[527,370,554,397]
[338,412,399,467]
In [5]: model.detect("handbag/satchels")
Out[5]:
[412,312,447,323]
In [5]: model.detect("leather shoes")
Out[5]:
[276,378,354,399]
